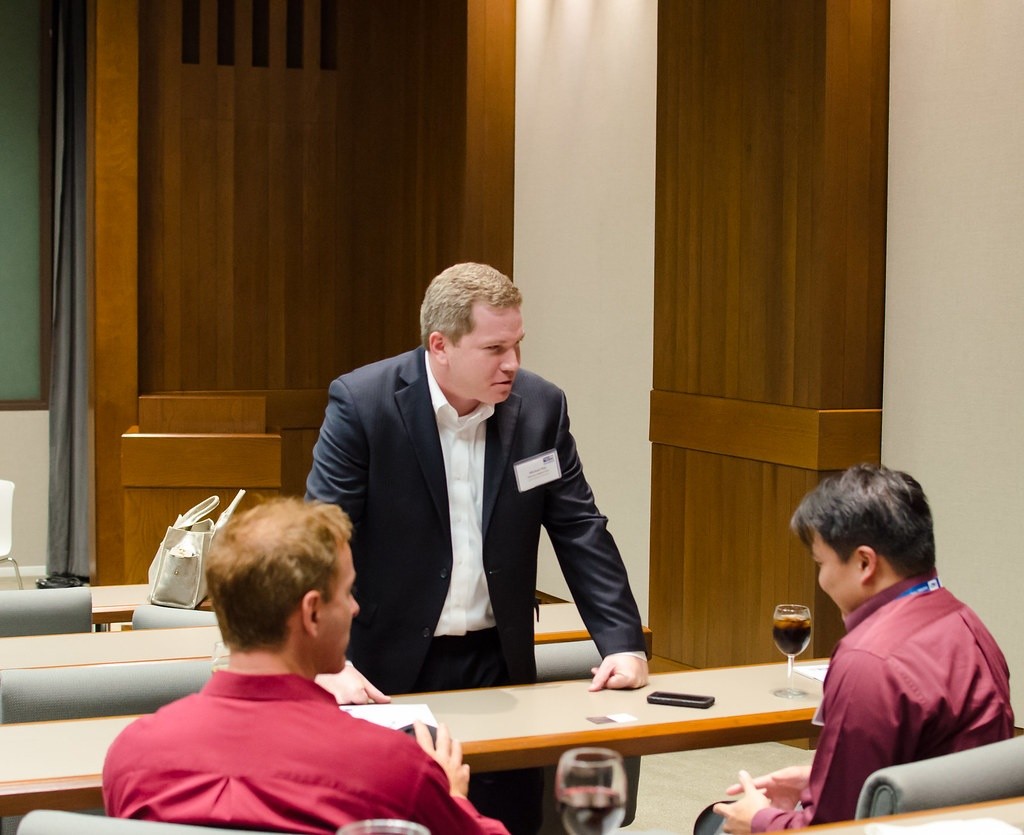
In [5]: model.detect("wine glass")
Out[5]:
[770,603,814,699]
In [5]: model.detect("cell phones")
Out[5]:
[647,691,714,708]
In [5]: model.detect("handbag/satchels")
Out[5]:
[148,489,245,608]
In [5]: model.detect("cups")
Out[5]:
[336,818,431,835]
[554,747,628,835]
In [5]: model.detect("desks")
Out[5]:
[0,657,834,817]
[0,603,653,681]
[88,585,214,625]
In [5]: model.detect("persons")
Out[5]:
[101,496,508,835]
[304,262,649,835]
[693,462,1014,835]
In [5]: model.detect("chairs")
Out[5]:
[0,585,1024,835]
